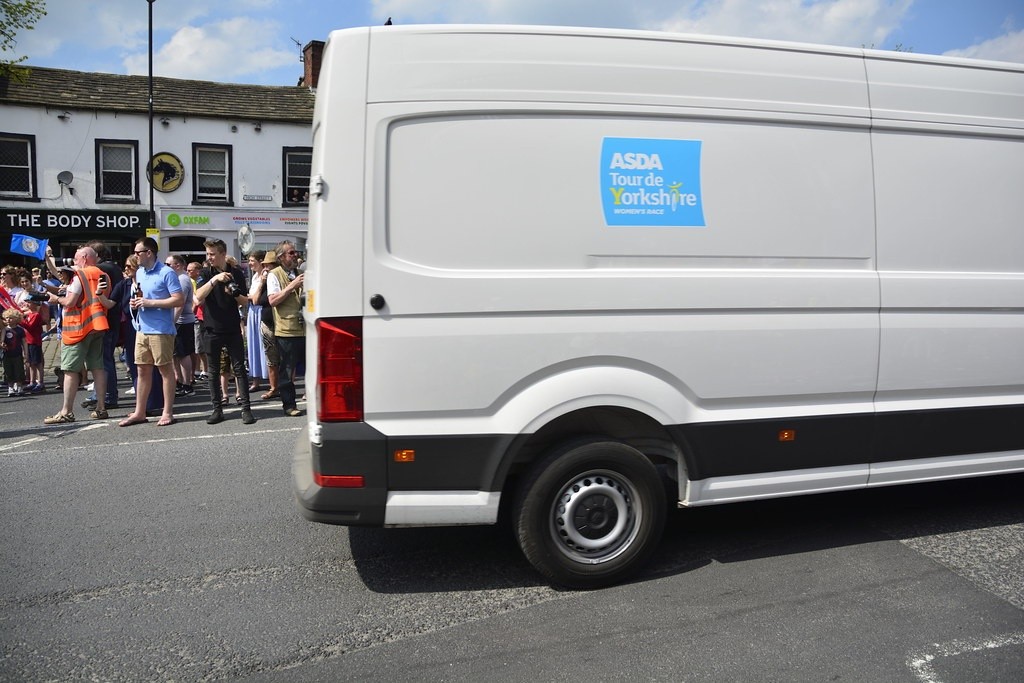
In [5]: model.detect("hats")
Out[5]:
[261,251,277,264]
[24,294,42,307]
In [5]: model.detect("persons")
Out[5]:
[267,240,305,416]
[1,308,28,397]
[303,192,309,202]
[119,237,184,427]
[0,240,307,411]
[96,254,156,417]
[44,247,111,424]
[246,249,268,391]
[289,190,304,202]
[195,238,255,424]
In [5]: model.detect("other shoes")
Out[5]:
[221,397,229,406]
[125,386,136,394]
[174,380,196,399]
[193,370,208,383]
[7,377,45,396]
[235,395,243,405]
[83,382,94,392]
[248,384,261,393]
[87,401,119,411]
[282,405,302,416]
[80,399,98,409]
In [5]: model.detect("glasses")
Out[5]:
[57,271,64,274]
[124,265,132,268]
[164,262,171,267]
[0,272,7,276]
[277,251,298,257]
[134,249,149,256]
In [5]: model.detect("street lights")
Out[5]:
[144,0,160,228]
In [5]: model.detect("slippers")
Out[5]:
[118,418,148,427]
[157,418,178,426]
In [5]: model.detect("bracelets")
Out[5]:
[210,279,213,286]
[57,298,60,304]
[43,283,46,287]
[95,292,102,295]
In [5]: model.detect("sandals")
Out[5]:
[260,388,280,399]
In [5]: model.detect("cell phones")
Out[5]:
[100,274,106,289]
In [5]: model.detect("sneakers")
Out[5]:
[206,409,225,424]
[88,408,108,420]
[43,411,75,423]
[240,408,254,424]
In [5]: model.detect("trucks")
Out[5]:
[286,20,1024,589]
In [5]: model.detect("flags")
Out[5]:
[11,234,48,260]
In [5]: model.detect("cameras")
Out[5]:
[29,292,66,308]
[225,278,241,297]
